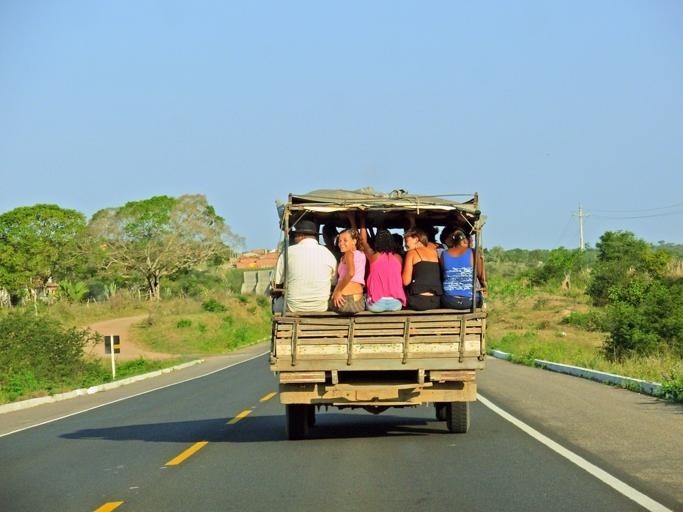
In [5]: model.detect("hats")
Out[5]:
[291,221,322,235]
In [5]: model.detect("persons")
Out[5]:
[271,211,483,316]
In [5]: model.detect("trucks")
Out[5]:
[263,184,489,442]
